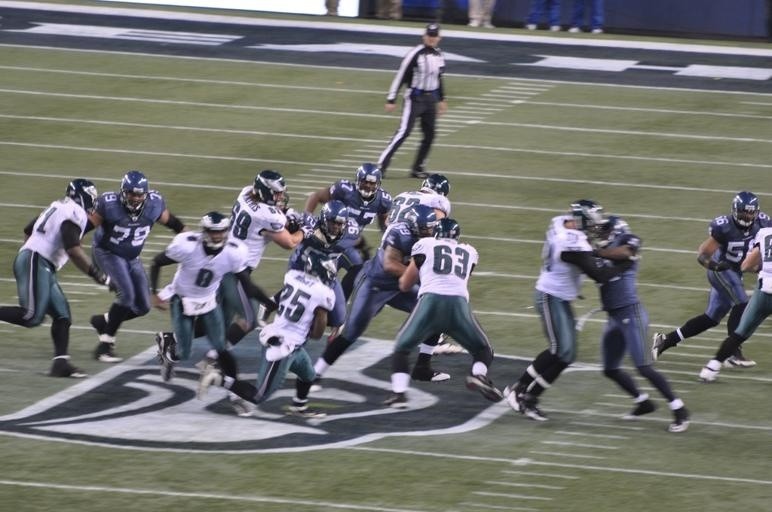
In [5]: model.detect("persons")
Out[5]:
[564,0,609,34]
[503,198,692,432]
[376,21,449,184]
[372,0,405,21]
[525,0,564,33]
[464,0,499,30]
[0,178,111,378]
[150,164,502,417]
[82,169,184,363]
[327,0,341,17]
[651,192,772,380]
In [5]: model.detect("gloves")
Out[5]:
[300,225,314,240]
[106,276,120,296]
[717,260,731,272]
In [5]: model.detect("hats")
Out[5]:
[426,24,439,37]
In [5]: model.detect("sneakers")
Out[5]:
[50,360,88,379]
[466,374,504,402]
[378,392,407,408]
[725,357,757,368]
[160,344,176,381]
[92,347,123,364]
[286,405,327,418]
[508,390,548,421]
[90,314,117,350]
[503,384,513,398]
[652,333,667,361]
[412,170,429,178]
[699,367,720,382]
[197,365,221,400]
[669,413,693,433]
[153,331,179,364]
[412,369,451,383]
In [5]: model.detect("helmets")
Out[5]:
[405,204,437,237]
[198,212,231,250]
[65,179,99,216]
[730,191,761,230]
[118,171,149,211]
[252,169,289,206]
[570,199,603,238]
[356,164,383,197]
[321,200,349,235]
[421,173,450,197]
[597,216,631,248]
[303,254,337,289]
[433,218,460,239]
